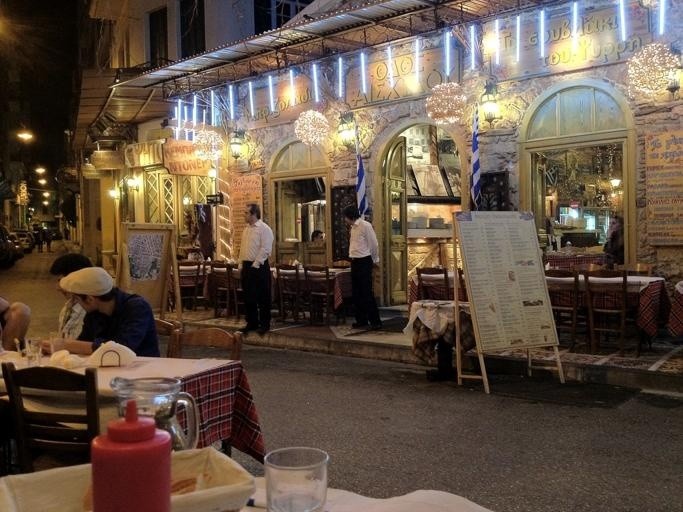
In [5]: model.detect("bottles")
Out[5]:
[89,397,173,512]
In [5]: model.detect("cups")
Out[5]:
[0,331,81,368]
[263,446,329,511]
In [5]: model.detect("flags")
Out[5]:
[470,114,482,210]
[355,155,368,216]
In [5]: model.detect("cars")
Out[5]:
[0,223,62,271]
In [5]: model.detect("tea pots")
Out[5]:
[106,376,202,449]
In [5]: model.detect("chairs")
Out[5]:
[154,317,182,358]
[1,360,100,474]
[583,269,637,355]
[569,262,609,277]
[457,267,468,302]
[416,266,450,300]
[170,327,244,360]
[166,259,349,328]
[613,262,657,277]
[545,269,589,345]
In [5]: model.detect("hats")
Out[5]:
[58,266,114,297]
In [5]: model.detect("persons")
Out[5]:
[64,225,69,240]
[342,205,383,330]
[38,227,52,253]
[312,230,324,242]
[49,253,95,337]
[0,297,31,351]
[603,214,624,269]
[37,266,159,358]
[238,204,274,333]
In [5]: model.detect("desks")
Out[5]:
[561,229,602,247]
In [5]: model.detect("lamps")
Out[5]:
[665,78,680,99]
[229,129,251,160]
[425,81,469,126]
[626,42,681,95]
[335,110,354,133]
[193,130,226,162]
[294,109,330,146]
[479,74,504,128]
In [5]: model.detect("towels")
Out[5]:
[89,341,137,367]
